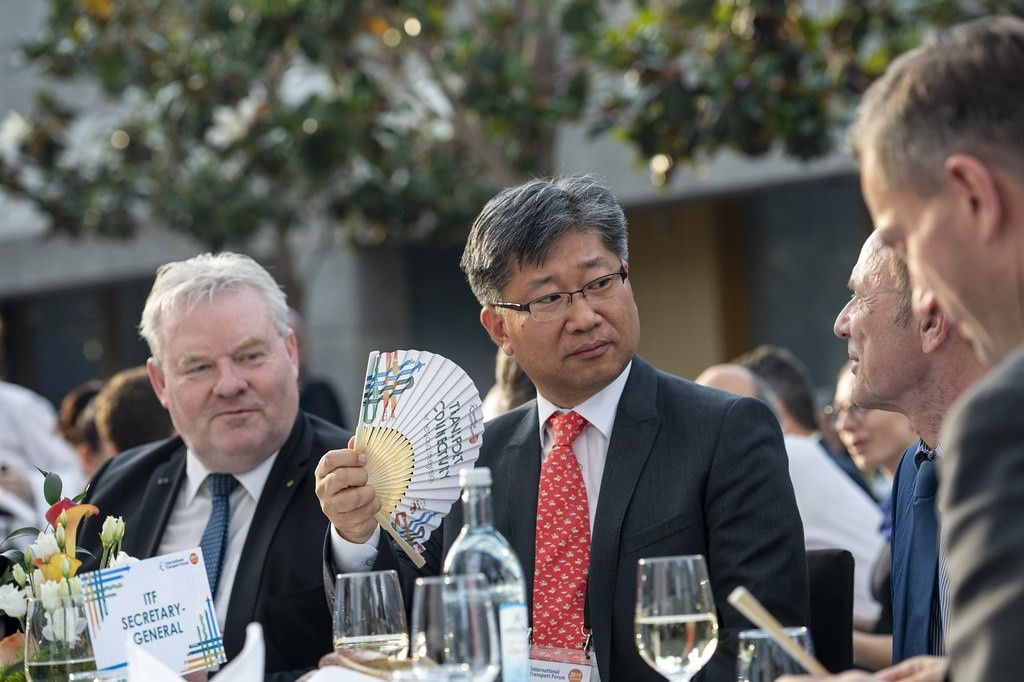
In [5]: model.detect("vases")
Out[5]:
[23,591,107,682]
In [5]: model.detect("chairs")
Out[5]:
[808,548,856,674]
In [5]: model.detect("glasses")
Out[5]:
[489,265,627,322]
[825,402,867,431]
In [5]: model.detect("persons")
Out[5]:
[0,363,345,568]
[0,252,354,682]
[311,177,810,682]
[480,14,1024,682]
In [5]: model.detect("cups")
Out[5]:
[332,571,407,660]
[26,596,97,681]
[411,575,501,682]
[738,629,812,682]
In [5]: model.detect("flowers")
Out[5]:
[0,462,140,645]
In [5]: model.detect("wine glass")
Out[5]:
[632,554,718,682]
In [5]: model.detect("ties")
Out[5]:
[198,473,240,603]
[902,459,941,661]
[532,411,592,665]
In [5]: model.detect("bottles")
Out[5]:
[443,466,529,682]
